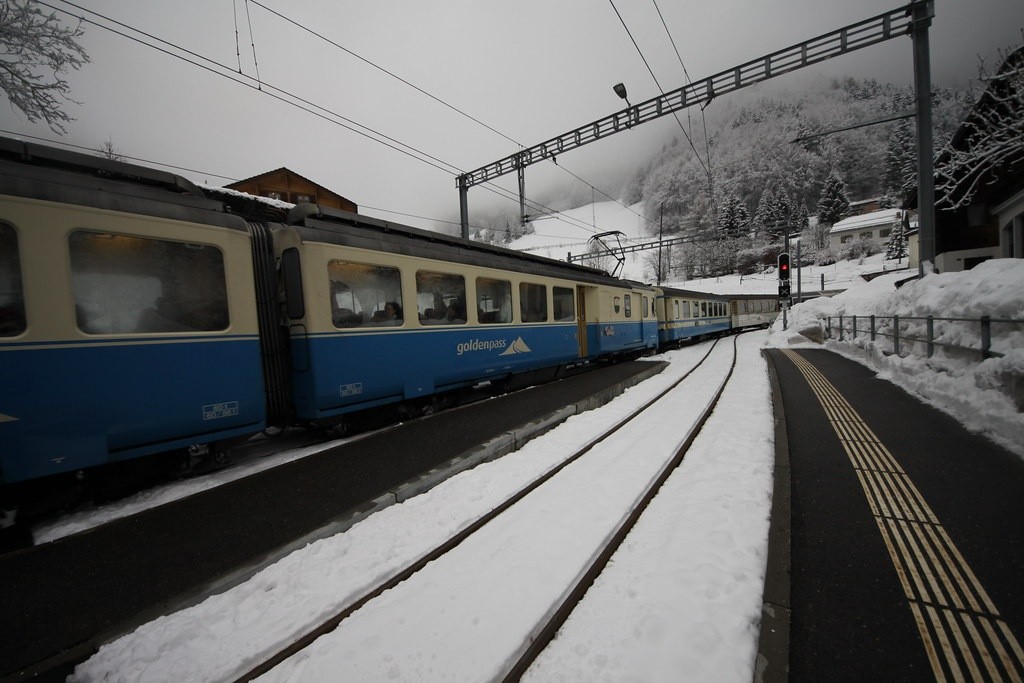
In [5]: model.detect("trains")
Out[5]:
[0,136,778,503]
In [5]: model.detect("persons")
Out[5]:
[702,306,727,316]
[419,294,466,324]
[521,306,546,322]
[358,300,404,326]
[136,254,230,334]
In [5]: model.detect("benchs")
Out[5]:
[372,310,403,322]
[481,311,500,322]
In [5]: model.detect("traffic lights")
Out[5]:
[778,252,791,281]
